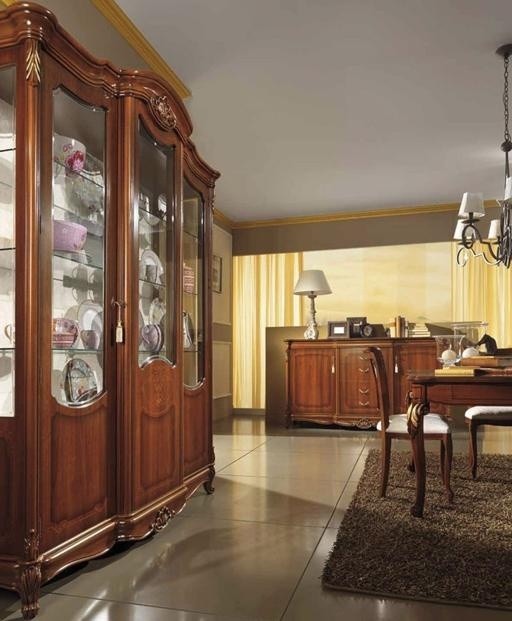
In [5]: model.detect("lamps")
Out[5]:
[293,269,332,341]
[453,39,512,270]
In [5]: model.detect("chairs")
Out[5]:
[365,344,454,502]
[464,404,512,480]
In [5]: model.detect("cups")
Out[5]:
[431,335,465,371]
[145,265,158,283]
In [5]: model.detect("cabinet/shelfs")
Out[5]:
[280,336,457,430]
[1,0,221,621]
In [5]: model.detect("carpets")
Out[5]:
[319,449,512,612]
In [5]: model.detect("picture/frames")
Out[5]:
[211,254,224,293]
[346,316,367,337]
[326,321,351,341]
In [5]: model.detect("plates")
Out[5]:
[140,248,164,285]
[141,209,161,225]
[140,324,165,354]
[183,311,194,349]
[52,318,78,343]
[59,358,97,407]
[149,298,166,323]
[77,301,103,330]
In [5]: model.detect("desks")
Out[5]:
[405,373,512,518]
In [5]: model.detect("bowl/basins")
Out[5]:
[51,332,75,349]
[182,266,195,293]
[53,136,87,184]
[54,218,88,250]
